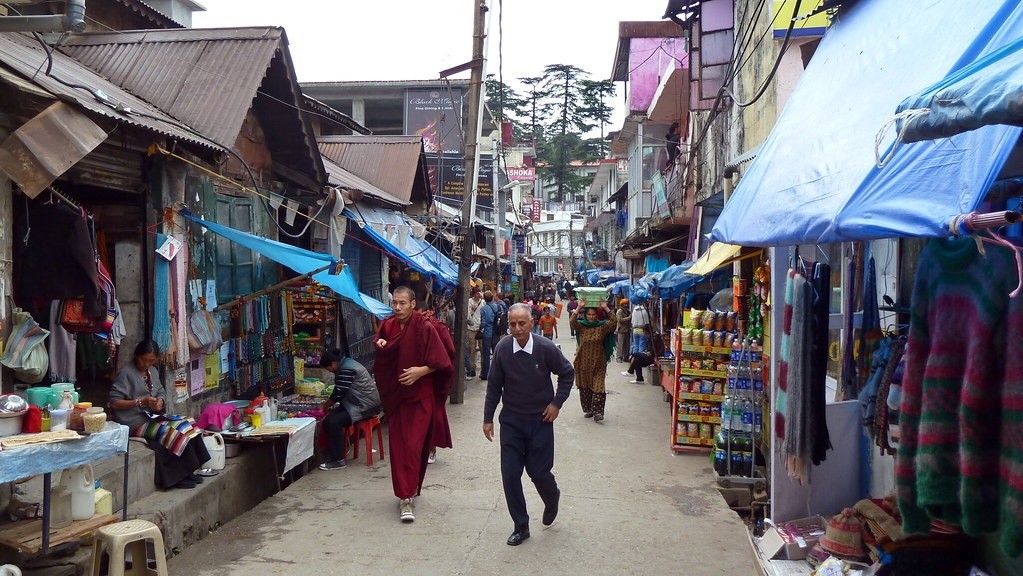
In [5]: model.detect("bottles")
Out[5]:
[26,383,107,434]
[254,396,278,424]
[297,377,325,396]
[48,486,73,528]
[93,481,112,516]
[710,338,763,477]
[59,464,95,521]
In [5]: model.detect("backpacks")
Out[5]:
[494,304,507,336]
[530,305,540,325]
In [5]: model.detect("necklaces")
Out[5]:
[141,369,153,391]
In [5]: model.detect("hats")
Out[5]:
[819,507,863,557]
[620,299,628,305]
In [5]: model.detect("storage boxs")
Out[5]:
[576,287,608,308]
[757,513,830,559]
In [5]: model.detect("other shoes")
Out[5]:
[427,449,437,463]
[174,479,195,490]
[621,371,633,377]
[466,377,471,380]
[630,380,645,384]
[479,372,487,381]
[470,371,476,377]
[593,413,604,420]
[186,471,203,484]
[319,459,347,470]
[584,411,592,417]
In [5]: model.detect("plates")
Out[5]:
[223,400,251,408]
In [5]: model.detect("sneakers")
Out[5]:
[398,497,414,520]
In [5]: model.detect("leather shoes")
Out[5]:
[508,528,530,545]
[543,489,560,525]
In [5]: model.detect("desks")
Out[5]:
[221,395,340,492]
[0,421,130,556]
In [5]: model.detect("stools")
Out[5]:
[88,519,169,576]
[354,416,385,466]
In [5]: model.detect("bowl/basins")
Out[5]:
[0,394,29,413]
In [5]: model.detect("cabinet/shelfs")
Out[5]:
[671,324,732,455]
[292,294,337,369]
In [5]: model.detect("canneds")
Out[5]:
[69,402,108,433]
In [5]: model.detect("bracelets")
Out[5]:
[135,398,142,407]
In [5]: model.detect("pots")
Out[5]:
[209,413,234,431]
[226,444,240,457]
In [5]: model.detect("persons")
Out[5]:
[447,298,474,380]
[629,303,650,363]
[538,306,558,341]
[524,272,611,346]
[483,303,575,546]
[621,324,665,384]
[570,298,617,421]
[318,348,382,470]
[614,298,632,363]
[414,303,453,463]
[373,285,455,522]
[467,287,486,377]
[479,290,512,379]
[109,339,211,489]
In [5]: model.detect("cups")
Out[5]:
[251,415,261,426]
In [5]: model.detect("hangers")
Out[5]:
[789,238,876,286]
[146,206,191,238]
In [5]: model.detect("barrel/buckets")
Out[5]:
[575,291,609,307]
[202,432,225,470]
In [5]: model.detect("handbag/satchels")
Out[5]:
[0,295,51,384]
[186,310,224,355]
